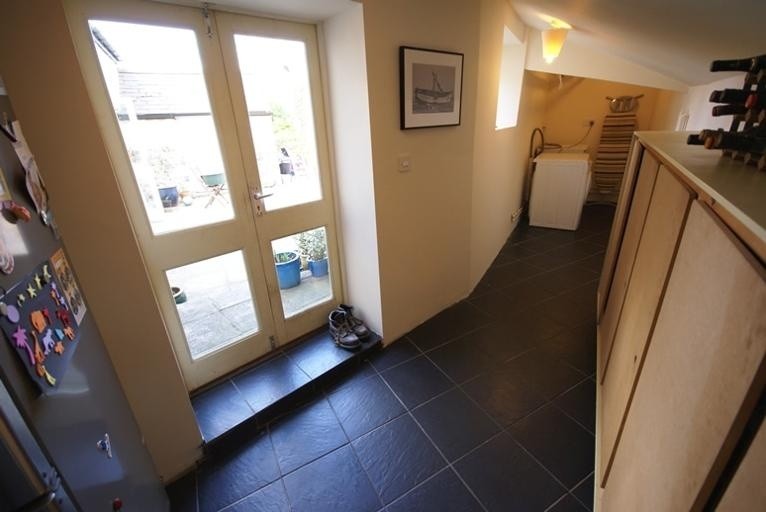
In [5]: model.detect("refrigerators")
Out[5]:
[0,88,172,512]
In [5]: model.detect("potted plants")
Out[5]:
[273,228,328,287]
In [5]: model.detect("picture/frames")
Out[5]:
[397,44,463,132]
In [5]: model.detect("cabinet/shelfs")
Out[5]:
[594,133,765,510]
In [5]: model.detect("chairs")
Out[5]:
[200,172,228,208]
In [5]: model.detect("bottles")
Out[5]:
[688,56,766,157]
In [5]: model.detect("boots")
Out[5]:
[329,311,361,348]
[338,304,369,339]
